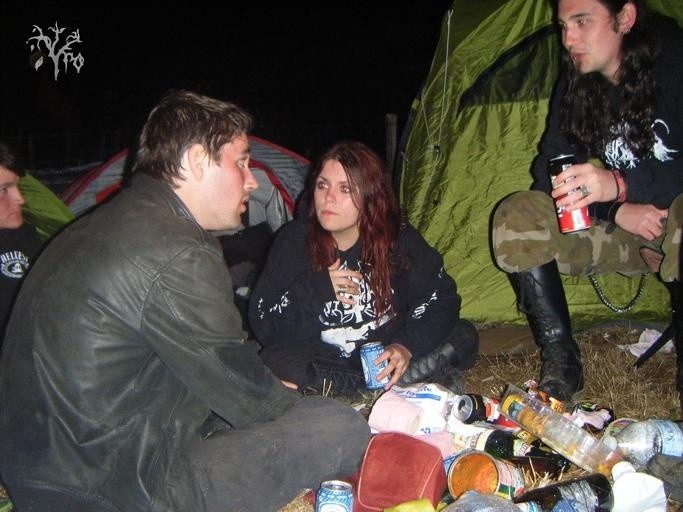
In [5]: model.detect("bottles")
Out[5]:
[602,418,683,468]
[509,471,614,512]
[474,428,570,480]
[609,459,669,512]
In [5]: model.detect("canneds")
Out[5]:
[547,154,592,234]
[453,393,504,424]
[447,451,526,502]
[360,341,392,389]
[316,481,354,512]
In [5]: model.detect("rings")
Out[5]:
[339,287,347,293]
[580,186,589,197]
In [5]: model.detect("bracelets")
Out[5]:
[611,170,621,200]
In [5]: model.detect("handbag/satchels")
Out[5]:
[354,432,447,512]
[648,454,683,503]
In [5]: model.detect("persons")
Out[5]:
[248,139,479,399]
[0,87,372,512]
[490,1,683,408]
[0,146,44,344]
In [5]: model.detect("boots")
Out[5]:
[507,259,585,404]
[301,342,465,399]
